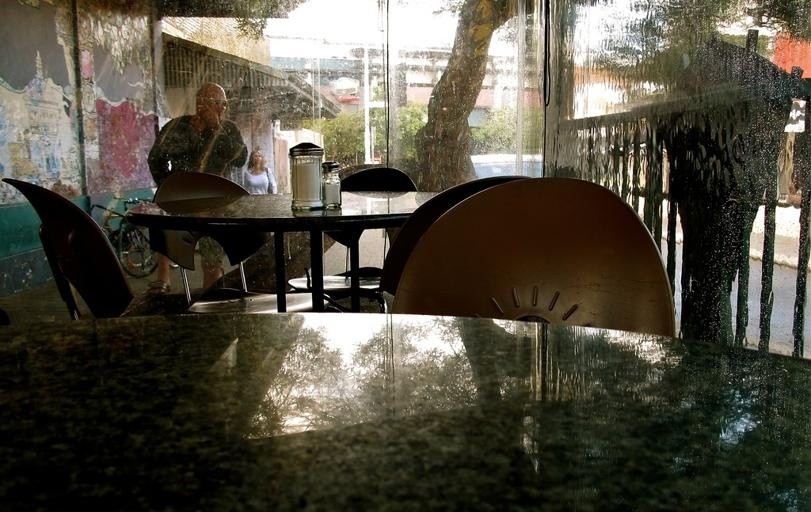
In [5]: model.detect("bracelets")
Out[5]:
[210,121,219,132]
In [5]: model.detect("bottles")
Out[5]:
[290,142,322,210]
[322,162,342,209]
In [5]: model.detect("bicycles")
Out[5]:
[88,197,181,277]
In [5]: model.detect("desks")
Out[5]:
[3,309,811,512]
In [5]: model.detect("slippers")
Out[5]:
[146,279,170,294]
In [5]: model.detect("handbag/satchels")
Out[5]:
[265,168,273,194]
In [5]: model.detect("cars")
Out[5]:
[472,154,545,178]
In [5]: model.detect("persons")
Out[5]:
[241,149,276,198]
[145,79,248,292]
[660,37,781,344]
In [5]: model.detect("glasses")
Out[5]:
[203,99,228,108]
